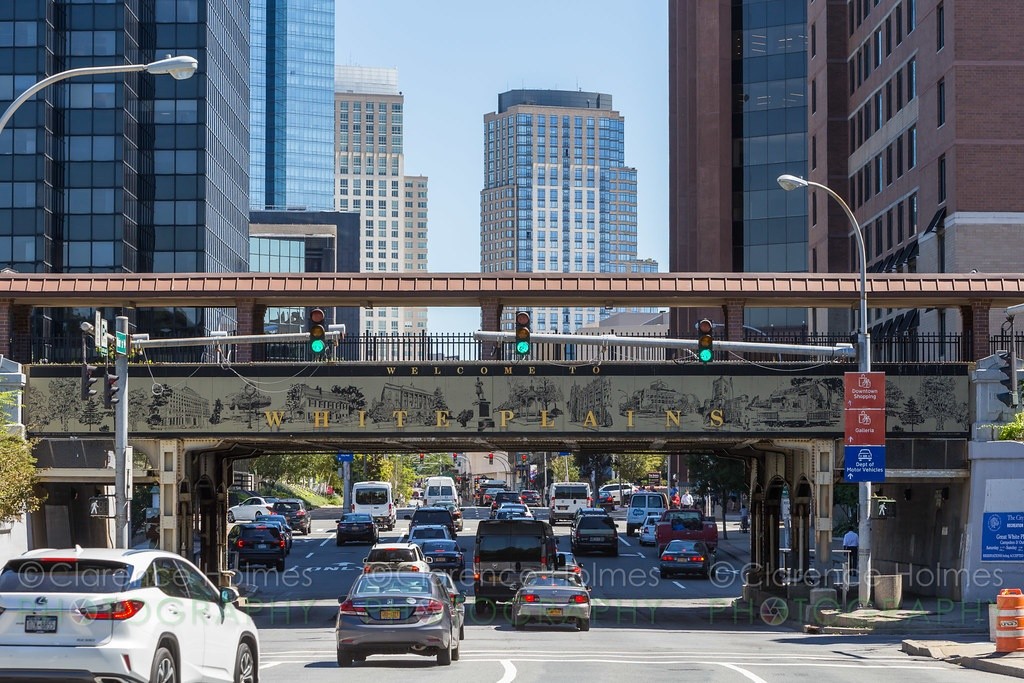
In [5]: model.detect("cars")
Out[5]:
[335,513,381,546]
[335,572,466,668]
[228,496,312,573]
[511,571,592,631]
[432,571,468,639]
[402,476,718,557]
[419,539,468,581]
[658,539,718,579]
[556,551,585,578]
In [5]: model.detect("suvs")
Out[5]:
[0,543,261,683]
[363,542,433,572]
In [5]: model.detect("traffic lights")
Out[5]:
[88,497,116,517]
[82,365,98,401]
[697,319,714,363]
[872,499,897,519]
[996,352,1019,409]
[103,374,120,410]
[420,453,424,464]
[489,454,493,465]
[522,455,527,465]
[515,310,531,355]
[309,309,326,354]
[453,453,458,463]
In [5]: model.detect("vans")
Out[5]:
[350,481,399,531]
[472,518,566,616]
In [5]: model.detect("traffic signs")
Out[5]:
[844,372,886,483]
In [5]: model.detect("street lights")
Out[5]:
[777,174,877,618]
[484,456,507,483]
